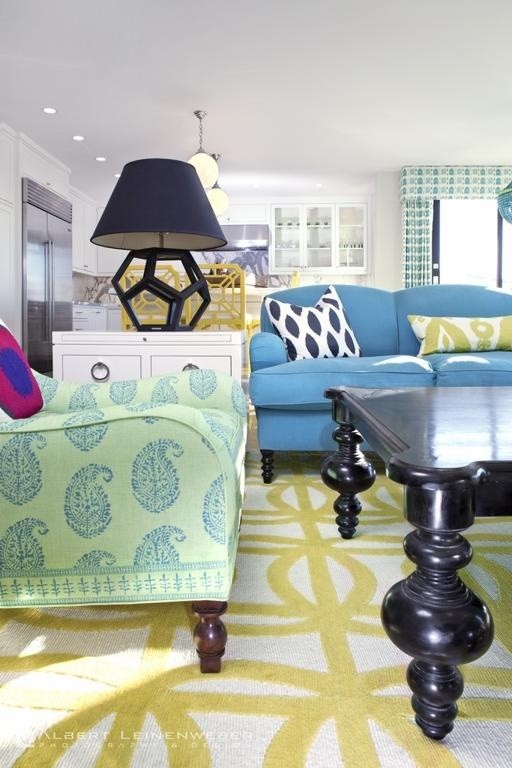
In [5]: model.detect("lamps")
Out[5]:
[206,154,229,216]
[90,158,227,331]
[185,111,222,191]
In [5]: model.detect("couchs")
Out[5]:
[249,284,512,484]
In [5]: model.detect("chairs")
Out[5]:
[0,368,247,672]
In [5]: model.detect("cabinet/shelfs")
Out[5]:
[268,198,371,276]
[69,190,186,277]
[73,305,126,330]
[52,330,246,383]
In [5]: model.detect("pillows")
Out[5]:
[0,324,43,419]
[407,313,512,356]
[264,285,361,361]
